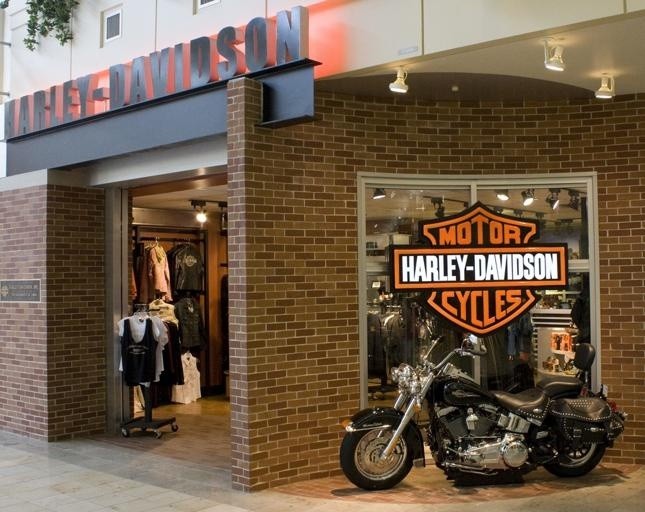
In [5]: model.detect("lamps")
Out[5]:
[388,65,410,94]
[372,188,386,200]
[423,195,546,230]
[594,73,617,100]
[495,187,586,210]
[542,38,566,72]
[191,200,228,224]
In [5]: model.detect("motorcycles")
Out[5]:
[340,332,628,490]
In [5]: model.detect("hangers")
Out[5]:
[133,234,201,364]
